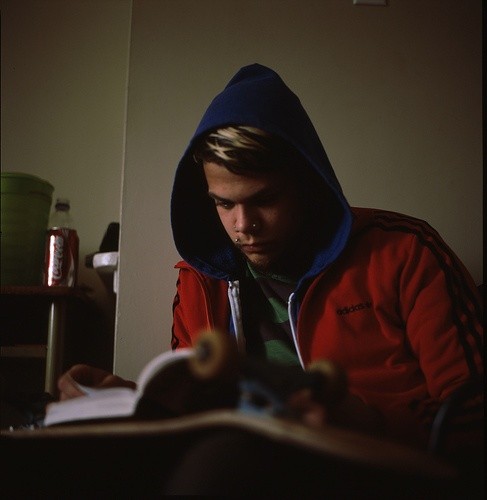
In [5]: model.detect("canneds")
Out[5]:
[43,226,78,289]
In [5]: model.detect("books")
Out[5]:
[42,347,194,427]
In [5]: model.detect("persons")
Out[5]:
[58,64,487,500]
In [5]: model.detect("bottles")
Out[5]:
[44,196,80,289]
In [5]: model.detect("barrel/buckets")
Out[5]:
[0,172,54,287]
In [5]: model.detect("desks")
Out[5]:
[0,284,96,397]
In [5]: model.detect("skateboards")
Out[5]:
[3,331,457,481]
